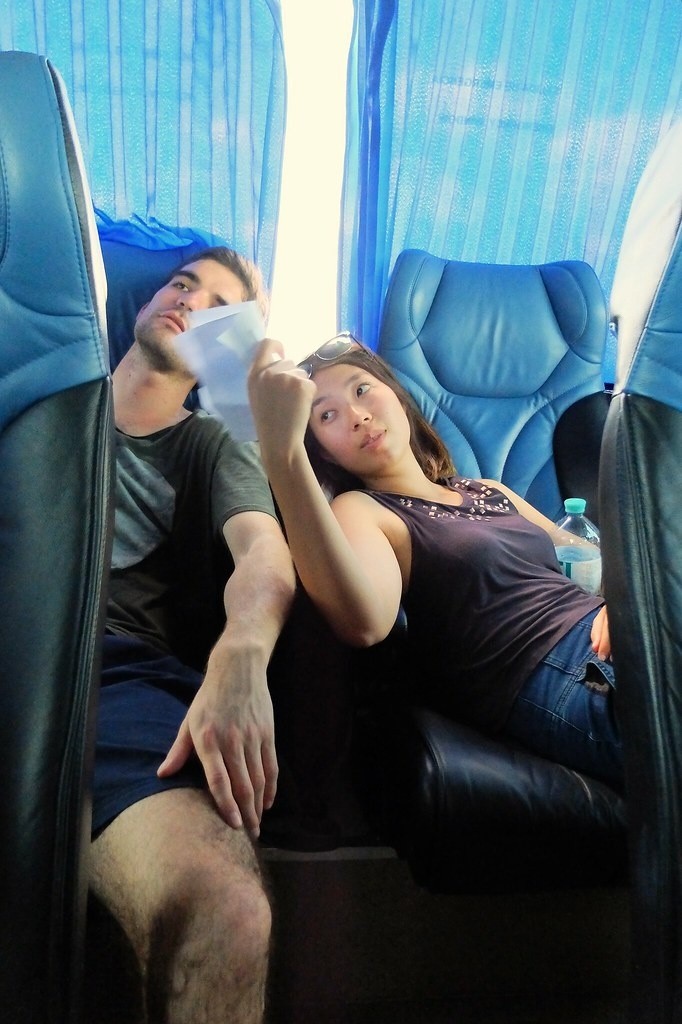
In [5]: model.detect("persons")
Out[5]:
[90,245,297,1024]
[246,329,627,791]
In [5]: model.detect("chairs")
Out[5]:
[386,250,638,880]
[96,222,231,376]
[599,121,682,1024]
[0,49,118,1024]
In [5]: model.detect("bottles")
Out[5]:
[551,498,602,597]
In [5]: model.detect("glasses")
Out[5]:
[295,331,379,379]
